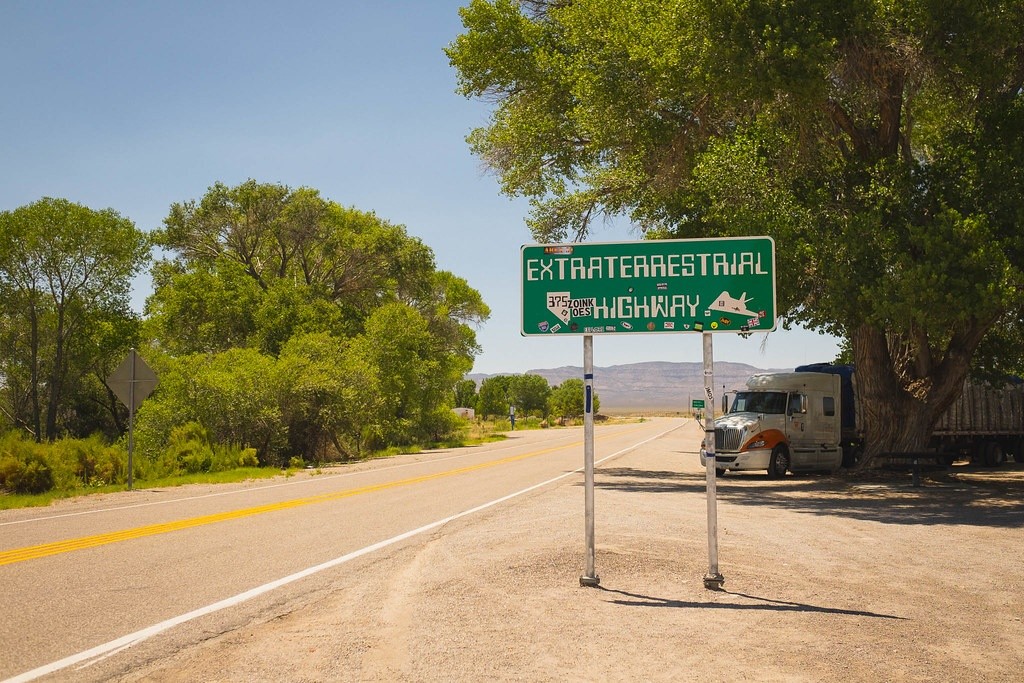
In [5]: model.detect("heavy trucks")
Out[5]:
[701,363,1024,480]
